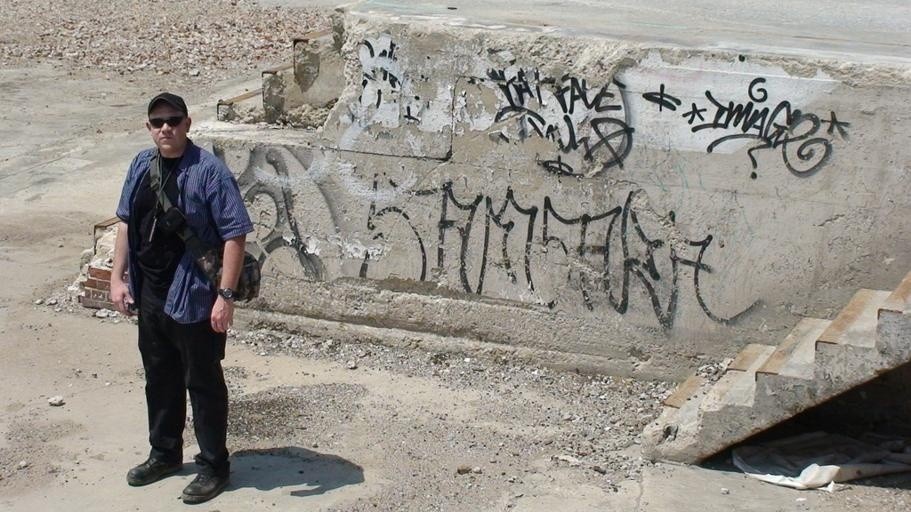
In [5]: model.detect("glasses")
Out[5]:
[148,117,183,128]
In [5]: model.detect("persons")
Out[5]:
[110,92,256,503]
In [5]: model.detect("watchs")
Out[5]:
[215,287,242,299]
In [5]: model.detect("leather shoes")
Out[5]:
[127,457,182,486]
[182,473,230,504]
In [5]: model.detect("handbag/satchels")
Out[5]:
[198,246,260,300]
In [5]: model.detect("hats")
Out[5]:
[147,92,187,116]
